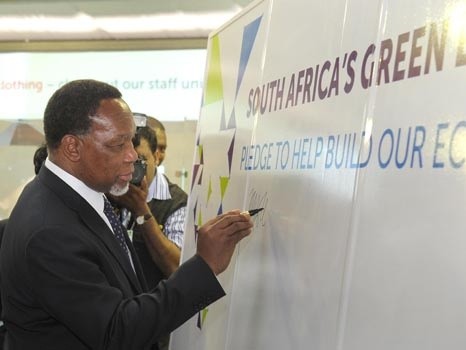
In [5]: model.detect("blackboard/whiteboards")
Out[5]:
[168,0,466,350]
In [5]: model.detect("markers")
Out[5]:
[240,208,264,216]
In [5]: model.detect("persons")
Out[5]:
[102,127,190,349]
[33,146,48,175]
[0,80,255,350]
[141,116,168,165]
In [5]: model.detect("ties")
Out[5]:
[103,194,129,263]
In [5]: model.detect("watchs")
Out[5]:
[135,213,152,225]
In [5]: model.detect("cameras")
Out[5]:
[129,159,147,186]
